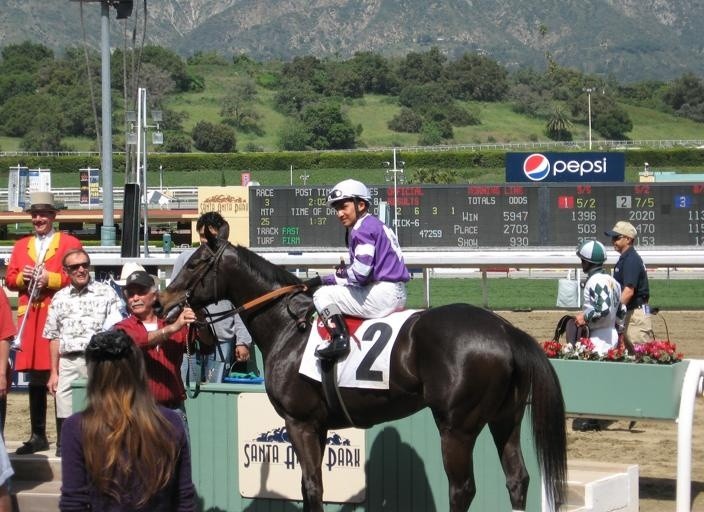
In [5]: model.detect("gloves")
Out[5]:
[332,255,347,278]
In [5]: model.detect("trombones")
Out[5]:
[10,236,53,352]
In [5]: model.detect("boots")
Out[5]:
[316,303,350,355]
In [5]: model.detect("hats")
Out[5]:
[602,219,638,241]
[22,191,60,214]
[121,270,155,289]
[110,261,147,287]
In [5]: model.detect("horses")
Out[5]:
[151,222,570,512]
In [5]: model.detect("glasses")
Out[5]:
[63,259,91,272]
[612,235,624,241]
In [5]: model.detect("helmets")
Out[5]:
[575,239,609,265]
[324,178,373,210]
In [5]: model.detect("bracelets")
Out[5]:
[161,326,168,342]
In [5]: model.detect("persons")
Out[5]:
[0,283,17,438]
[5,193,82,456]
[572,239,621,432]
[42,248,121,418]
[119,264,147,291]
[1,437,16,512]
[57,329,198,511]
[300,179,410,356]
[604,221,652,345]
[170,213,253,381]
[110,271,191,475]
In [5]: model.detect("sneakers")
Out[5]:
[17,432,50,454]
